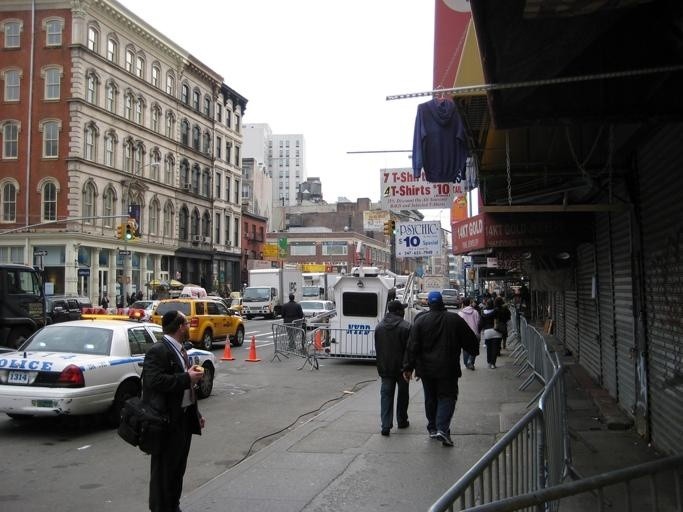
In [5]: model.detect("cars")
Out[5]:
[418,287,469,308]
[0,308,215,424]
[298,300,335,330]
[46,292,245,350]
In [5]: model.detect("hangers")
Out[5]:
[435,85,448,104]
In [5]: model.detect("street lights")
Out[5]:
[122,161,160,308]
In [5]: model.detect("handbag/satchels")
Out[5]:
[117,397,170,455]
[494,319,506,332]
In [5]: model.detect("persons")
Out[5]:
[279,293,304,350]
[100,289,158,309]
[398,290,481,447]
[136,309,207,512]
[373,298,414,437]
[455,282,530,370]
[7,273,26,294]
[217,281,248,298]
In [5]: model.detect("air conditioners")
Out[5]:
[192,235,203,244]
[183,183,192,191]
[244,249,249,256]
[244,232,248,238]
[225,240,231,247]
[226,140,235,148]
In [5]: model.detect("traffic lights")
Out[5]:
[125,219,133,241]
[116,226,123,241]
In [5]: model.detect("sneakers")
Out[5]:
[382,430,389,435]
[436,431,453,445]
[399,422,409,428]
[430,430,437,438]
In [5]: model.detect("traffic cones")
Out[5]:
[245,336,262,363]
[221,334,235,360]
[311,331,325,351]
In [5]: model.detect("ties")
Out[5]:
[181,346,196,401]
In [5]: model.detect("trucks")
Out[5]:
[385,274,420,309]
[1,263,46,346]
[303,273,342,305]
[242,268,302,319]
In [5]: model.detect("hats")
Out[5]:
[428,291,441,305]
[388,300,407,311]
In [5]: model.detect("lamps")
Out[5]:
[344,226,349,232]
